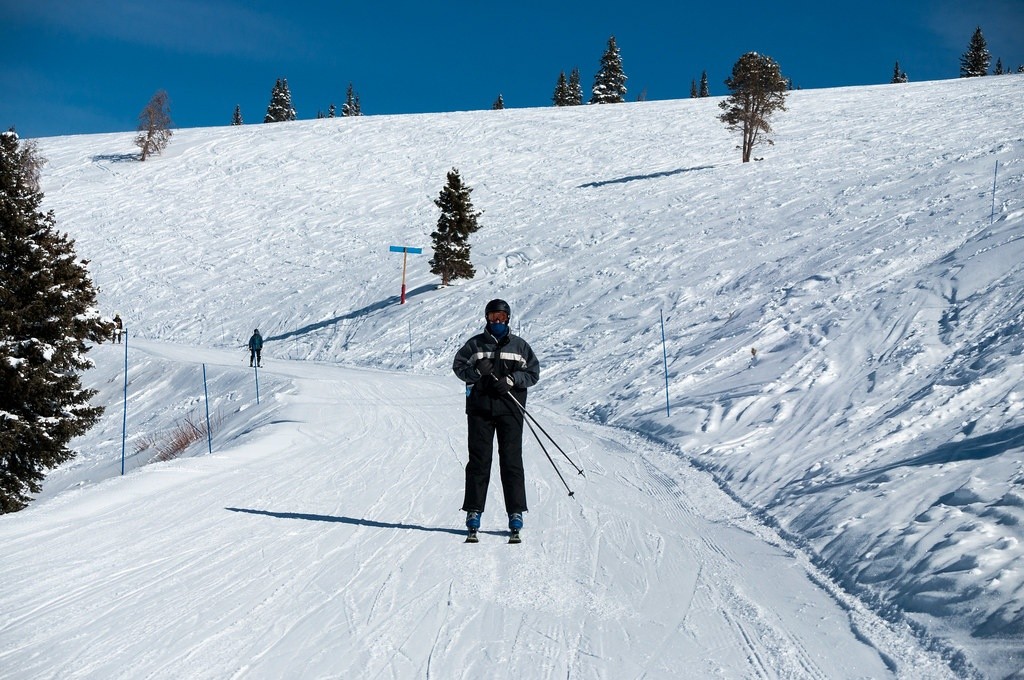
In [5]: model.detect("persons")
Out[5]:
[249,328,264,369]
[452,298,541,544]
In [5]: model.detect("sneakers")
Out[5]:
[465,508,482,529]
[508,509,524,529]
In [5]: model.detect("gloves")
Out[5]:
[494,374,515,395]
[475,357,494,376]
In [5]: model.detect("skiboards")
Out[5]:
[462,528,522,549]
[248,364,263,368]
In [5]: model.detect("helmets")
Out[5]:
[116,313,120,316]
[484,299,511,315]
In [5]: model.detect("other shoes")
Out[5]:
[112,341,115,343]
[118,341,121,344]
[250,363,253,367]
[257,362,260,367]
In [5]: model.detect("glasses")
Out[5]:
[486,311,509,322]
